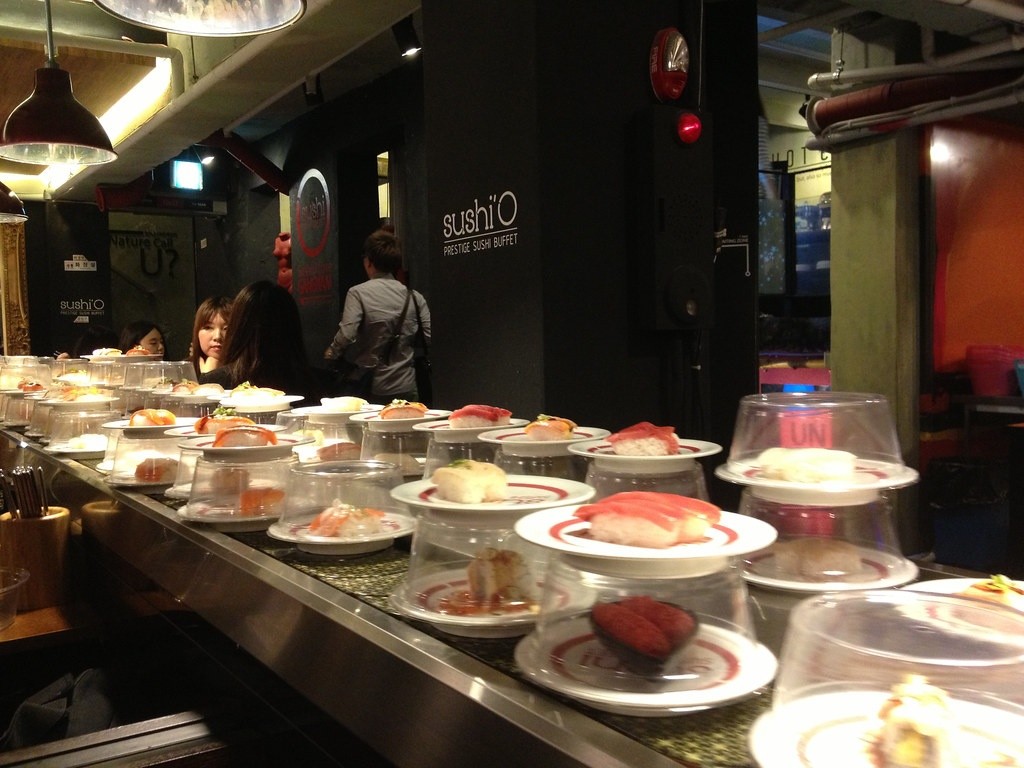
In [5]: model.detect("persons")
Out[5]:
[180,279,313,396]
[324,230,431,405]
[49,320,168,362]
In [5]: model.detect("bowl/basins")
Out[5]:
[0,566,31,631]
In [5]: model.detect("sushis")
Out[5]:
[17,345,152,398]
[129,381,581,600]
[757,446,1024,629]
[592,598,695,667]
[573,422,722,545]
[867,673,956,768]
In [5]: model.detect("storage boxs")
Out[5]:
[796,205,831,294]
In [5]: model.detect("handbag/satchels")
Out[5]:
[341,365,375,397]
[415,356,433,389]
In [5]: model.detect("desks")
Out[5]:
[0,598,107,657]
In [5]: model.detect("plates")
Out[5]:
[514,617,779,716]
[513,503,778,579]
[568,438,723,473]
[749,691,1024,767]
[0,388,203,429]
[715,458,920,506]
[267,513,417,554]
[896,578,1024,646]
[391,568,598,638]
[349,410,453,432]
[477,426,611,458]
[412,418,530,444]
[738,543,918,594]
[289,405,385,424]
[412,457,439,473]
[95,383,305,413]
[390,474,595,525]
[0,417,317,529]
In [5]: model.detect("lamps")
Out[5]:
[391,14,422,57]
[0,181,29,223]
[0,0,119,165]
[92,0,308,37]
